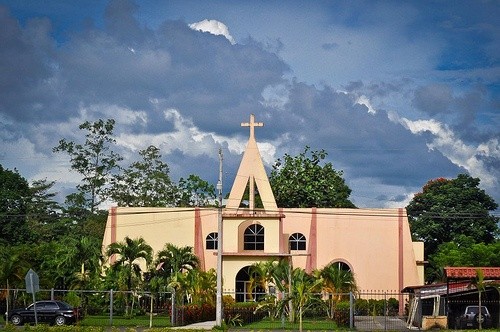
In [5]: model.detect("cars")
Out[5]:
[4,299,77,326]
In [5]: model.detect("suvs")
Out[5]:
[454,305,492,329]
